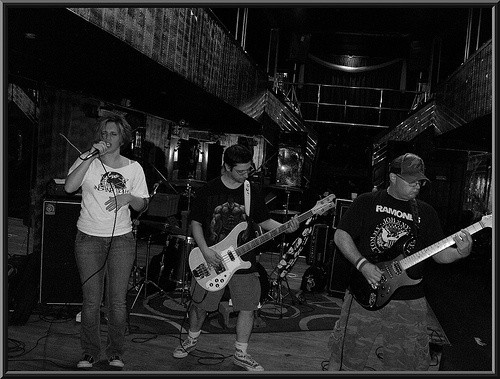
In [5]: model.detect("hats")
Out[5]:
[388,154,432,185]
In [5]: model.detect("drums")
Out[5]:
[307,224,341,267]
[161,234,200,284]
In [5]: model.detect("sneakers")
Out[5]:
[233,346,265,371]
[173,331,201,358]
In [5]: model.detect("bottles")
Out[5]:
[372,186,378,193]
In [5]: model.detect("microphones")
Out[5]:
[84,149,99,160]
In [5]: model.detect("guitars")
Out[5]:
[343,213,490,312]
[187,191,340,290]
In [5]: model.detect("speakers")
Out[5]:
[39,199,106,306]
[328,247,371,299]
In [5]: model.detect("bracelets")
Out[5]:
[356,257,368,271]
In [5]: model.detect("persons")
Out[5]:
[327,153,473,372]
[172,144,300,372]
[63,114,150,369]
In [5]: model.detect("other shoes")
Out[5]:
[106,355,124,367]
[77,354,100,367]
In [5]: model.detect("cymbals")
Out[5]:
[267,184,302,191]
[268,210,300,214]
[172,179,206,186]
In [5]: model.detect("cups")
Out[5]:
[351,192,358,200]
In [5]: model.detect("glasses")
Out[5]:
[233,165,255,175]
[396,174,426,186]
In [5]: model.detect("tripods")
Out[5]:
[128,234,188,317]
[268,192,296,319]
